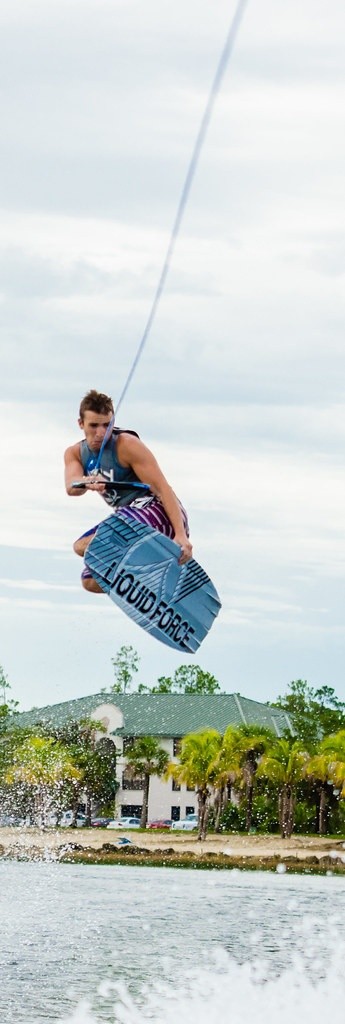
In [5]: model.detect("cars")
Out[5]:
[149,818,174,829]
[107,817,150,828]
[90,817,114,828]
[59,811,86,828]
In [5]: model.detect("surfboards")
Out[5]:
[81,512,224,655]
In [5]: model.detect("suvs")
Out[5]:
[172,813,198,830]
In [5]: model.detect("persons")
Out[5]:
[65,389,193,594]
